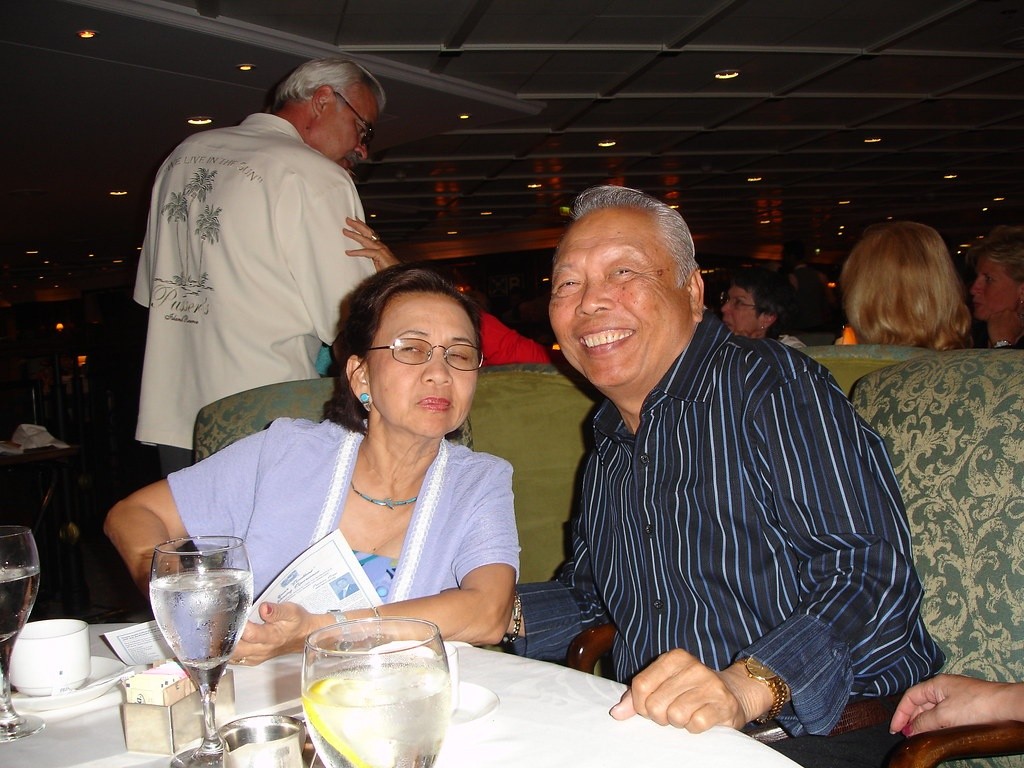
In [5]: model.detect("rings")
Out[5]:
[371,233,380,241]
[233,657,246,664]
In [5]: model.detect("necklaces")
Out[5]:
[351,481,417,509]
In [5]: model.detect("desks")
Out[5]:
[1,622,798,768]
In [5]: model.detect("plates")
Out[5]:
[452,681,500,724]
[9,657,125,709]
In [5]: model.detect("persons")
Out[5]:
[890,674,1024,737]
[967,226,1024,350]
[783,239,834,334]
[336,578,360,600]
[834,222,973,350]
[104,265,521,666]
[344,216,564,365]
[721,267,806,348]
[0,356,158,623]
[501,189,941,768]
[134,56,388,565]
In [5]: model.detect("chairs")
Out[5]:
[190,378,509,660]
[805,342,932,398]
[571,348,1023,768]
[464,361,611,678]
[882,720,1024,768]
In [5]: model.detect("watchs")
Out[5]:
[736,656,787,725]
[993,340,1011,347]
[327,610,354,652]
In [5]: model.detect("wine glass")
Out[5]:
[0,526,47,743]
[150,536,254,768]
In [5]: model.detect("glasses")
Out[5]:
[721,291,767,310]
[357,338,485,372]
[335,91,375,146]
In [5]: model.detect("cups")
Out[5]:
[218,618,460,768]
[10,619,89,695]
[368,639,458,715]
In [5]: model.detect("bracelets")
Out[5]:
[502,591,521,644]
[372,606,380,647]
